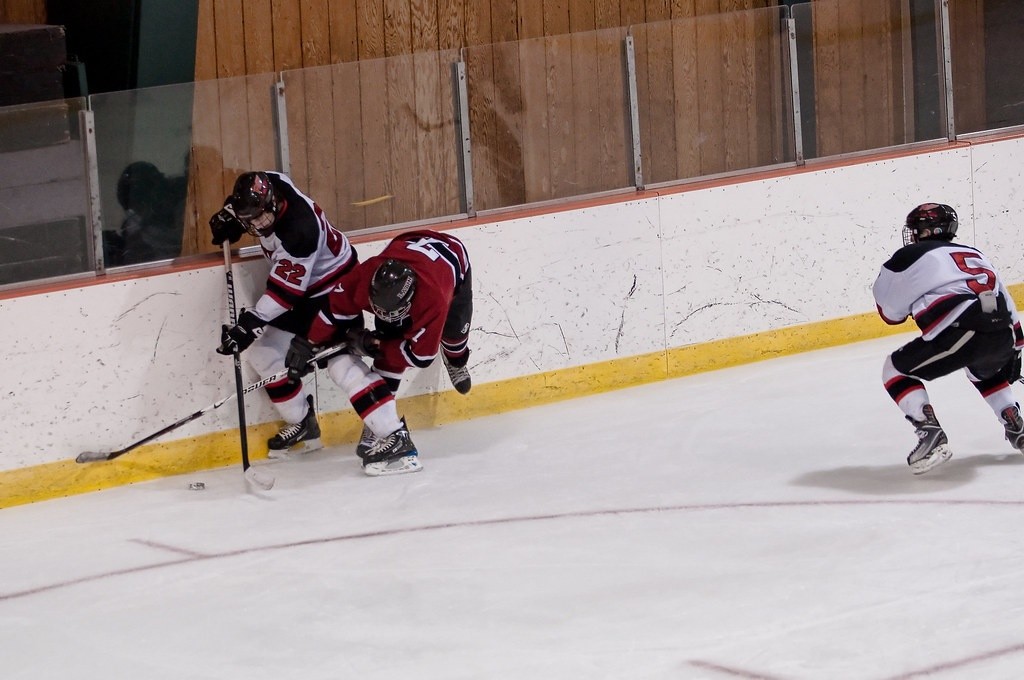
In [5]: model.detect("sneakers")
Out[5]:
[268,394,323,459]
[440,344,471,395]
[998,402,1024,455]
[905,405,952,475]
[362,415,423,476]
[356,423,380,459]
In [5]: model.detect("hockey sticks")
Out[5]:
[222,237,277,492]
[73,342,350,463]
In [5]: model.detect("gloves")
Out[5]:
[344,327,384,358]
[216,308,268,355]
[1001,347,1022,384]
[209,201,246,248]
[283,335,314,382]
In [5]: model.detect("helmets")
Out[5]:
[231,172,278,238]
[115,162,170,229]
[902,203,958,247]
[368,259,416,323]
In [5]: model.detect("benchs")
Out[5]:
[0,22,93,285]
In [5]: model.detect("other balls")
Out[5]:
[187,482,205,491]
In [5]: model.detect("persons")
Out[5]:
[872,203,1024,476]
[208,171,424,475]
[285,228,472,457]
[85,146,248,268]
